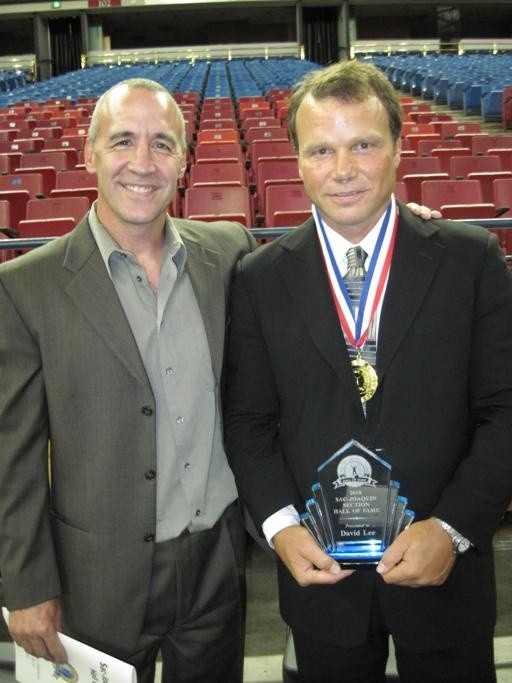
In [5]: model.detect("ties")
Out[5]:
[339,246,370,317]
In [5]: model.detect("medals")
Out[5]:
[350,353,381,405]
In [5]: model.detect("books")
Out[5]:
[2,604,141,683]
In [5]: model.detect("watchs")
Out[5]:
[433,517,471,555]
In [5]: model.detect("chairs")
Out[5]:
[0,53,512,269]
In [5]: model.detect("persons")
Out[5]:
[216,53,512,683]
[2,77,448,683]
